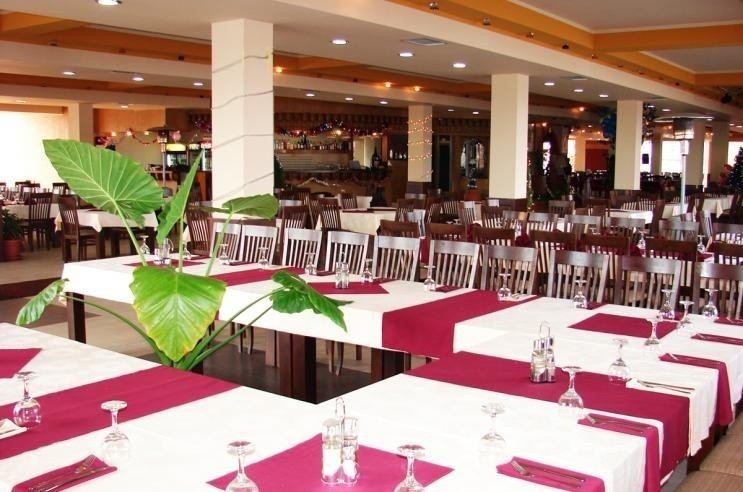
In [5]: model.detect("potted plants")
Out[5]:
[527,148,548,193]
[463,157,481,201]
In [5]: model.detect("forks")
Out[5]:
[511,458,581,489]
[27,454,96,490]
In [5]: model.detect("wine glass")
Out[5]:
[421,265,436,296]
[12,370,40,429]
[570,280,588,312]
[497,272,512,301]
[139,236,373,291]
[100,398,130,465]
[480,388,505,443]
[392,443,426,492]
[557,364,583,426]
[223,439,259,492]
[608,337,629,384]
[639,287,720,366]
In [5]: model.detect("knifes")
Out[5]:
[518,460,586,482]
[44,464,109,490]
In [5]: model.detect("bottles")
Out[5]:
[372,146,381,169]
[320,394,359,487]
[276,137,340,149]
[333,261,349,289]
[528,321,557,383]
